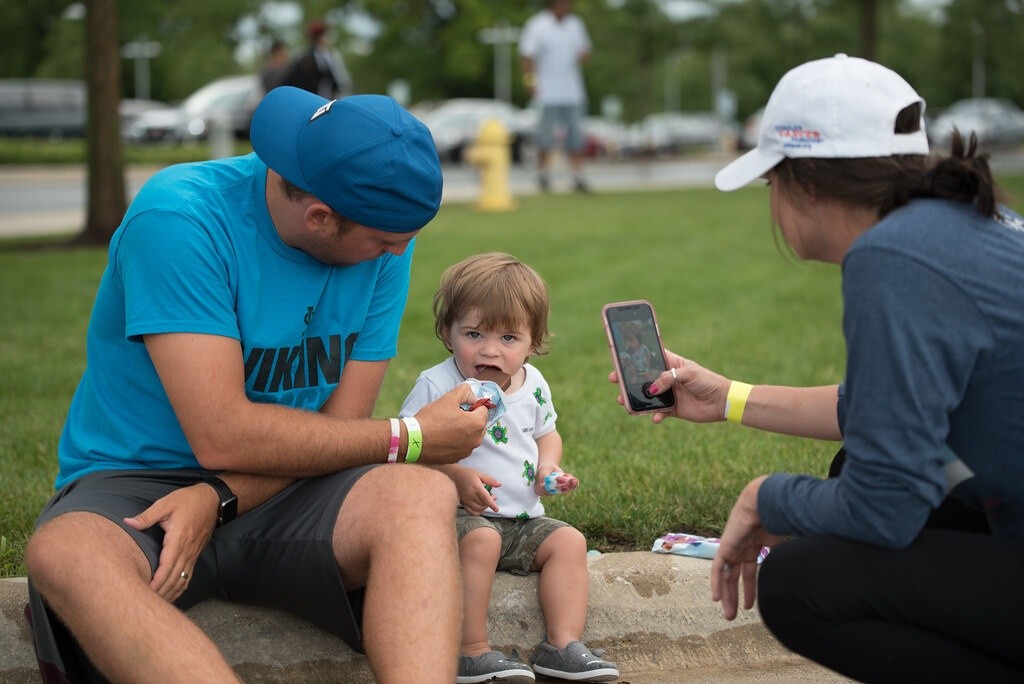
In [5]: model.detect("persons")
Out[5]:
[519,0,593,194]
[609,52,1024,684]
[24,84,487,684]
[619,323,656,383]
[400,252,621,684]
[260,20,352,102]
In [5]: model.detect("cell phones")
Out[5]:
[602,300,678,415]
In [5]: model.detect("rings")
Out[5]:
[671,367,678,384]
[180,572,188,579]
[723,563,732,572]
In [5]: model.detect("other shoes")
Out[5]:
[539,177,549,186]
[574,183,587,193]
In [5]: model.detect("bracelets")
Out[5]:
[724,379,753,424]
[387,417,400,465]
[402,417,422,464]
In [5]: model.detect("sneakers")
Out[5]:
[456,652,535,684]
[533,641,619,683]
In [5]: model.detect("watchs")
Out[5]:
[201,476,238,527]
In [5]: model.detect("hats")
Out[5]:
[250,87,443,233]
[305,20,328,39]
[714,53,930,191]
